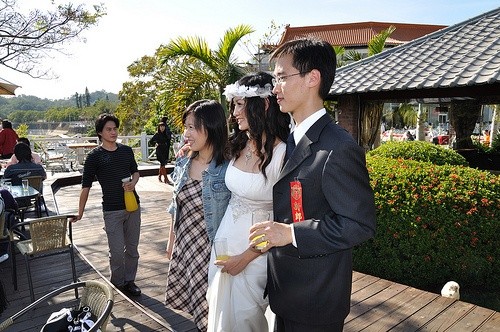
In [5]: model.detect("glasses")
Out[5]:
[272,68,322,87]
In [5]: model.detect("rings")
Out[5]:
[262,237,266,241]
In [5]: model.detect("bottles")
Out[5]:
[121,177,139,212]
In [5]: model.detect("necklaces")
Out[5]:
[245,139,265,165]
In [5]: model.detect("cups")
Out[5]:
[249,210,270,252]
[22,179,29,191]
[5,182,12,193]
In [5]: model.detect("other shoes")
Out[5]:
[0,254,9,263]
[116,286,127,295]
[125,282,141,295]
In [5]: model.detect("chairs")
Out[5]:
[0,143,114,332]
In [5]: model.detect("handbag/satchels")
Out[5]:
[41,306,96,332]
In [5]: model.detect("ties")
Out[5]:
[282,132,295,169]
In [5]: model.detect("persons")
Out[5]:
[380,116,490,149]
[249,37,383,332]
[0,72,300,332]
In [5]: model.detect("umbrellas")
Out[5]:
[0,77,22,95]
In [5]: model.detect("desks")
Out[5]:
[67,143,99,170]
[0,185,41,217]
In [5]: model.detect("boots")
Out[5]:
[159,171,162,182]
[162,170,173,184]
